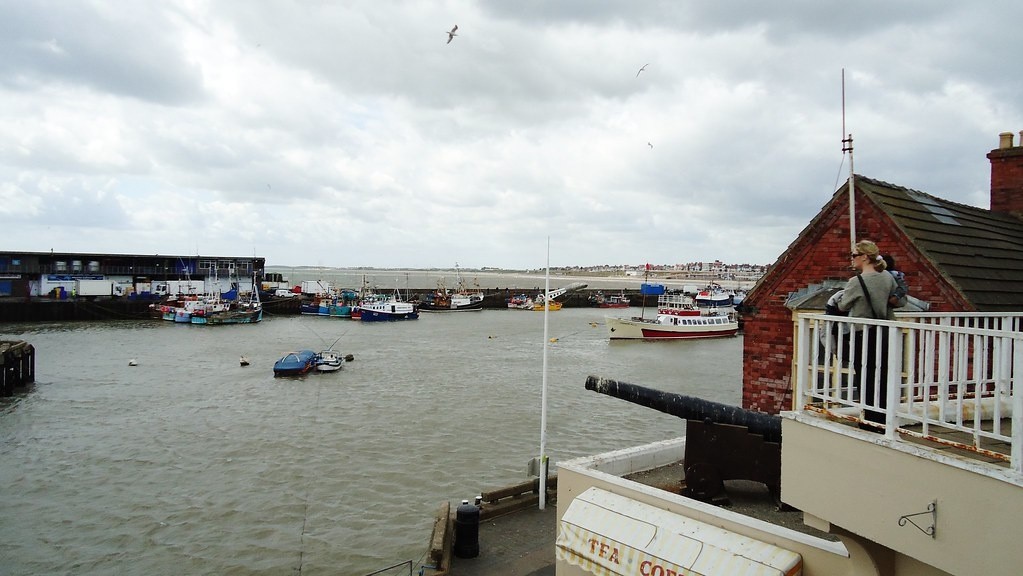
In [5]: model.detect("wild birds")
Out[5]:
[636,63,649,78]
[648,142,654,149]
[446,24,459,44]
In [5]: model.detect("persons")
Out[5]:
[836,240,909,434]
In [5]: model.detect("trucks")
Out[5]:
[76,279,123,299]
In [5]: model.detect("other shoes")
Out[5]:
[857,418,885,434]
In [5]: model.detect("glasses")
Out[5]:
[851,253,868,258]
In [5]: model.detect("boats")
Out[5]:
[149,249,740,324]
[603,268,740,339]
[306,326,349,375]
[274,349,316,378]
